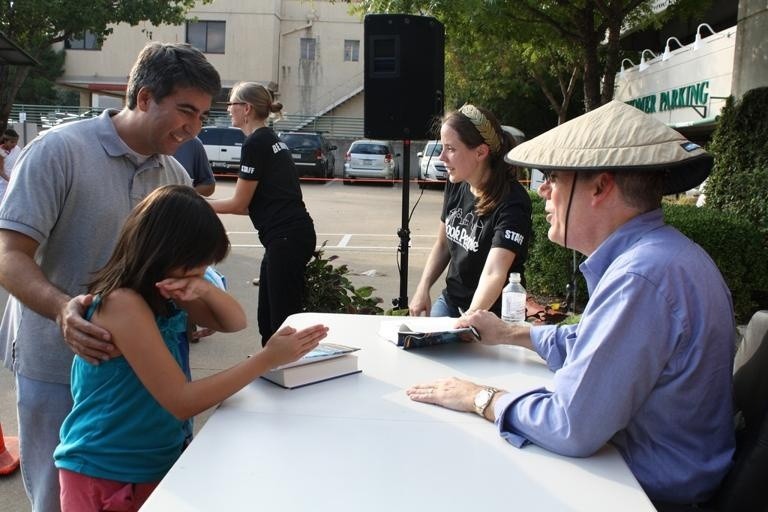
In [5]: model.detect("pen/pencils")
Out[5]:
[457,306,482,342]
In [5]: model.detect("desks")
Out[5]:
[134,309,659,511]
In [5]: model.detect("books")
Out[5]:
[247,345,364,390]
[375,314,476,348]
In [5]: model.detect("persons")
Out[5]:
[53,182,330,512]
[1,127,22,201]
[1,39,221,511]
[407,96,739,510]
[408,100,534,325]
[172,133,216,197]
[200,79,315,351]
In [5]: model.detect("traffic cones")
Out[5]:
[0,414,22,477]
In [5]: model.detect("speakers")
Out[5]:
[364,14,445,141]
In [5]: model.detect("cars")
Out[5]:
[7,111,102,130]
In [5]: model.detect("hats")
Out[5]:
[502,99,714,196]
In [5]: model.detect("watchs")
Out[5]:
[471,388,501,419]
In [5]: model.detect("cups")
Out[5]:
[12,112,26,124]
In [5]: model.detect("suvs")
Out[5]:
[277,130,338,184]
[196,123,246,181]
[416,140,449,190]
[344,138,402,187]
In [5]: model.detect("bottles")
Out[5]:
[499,273,529,349]
